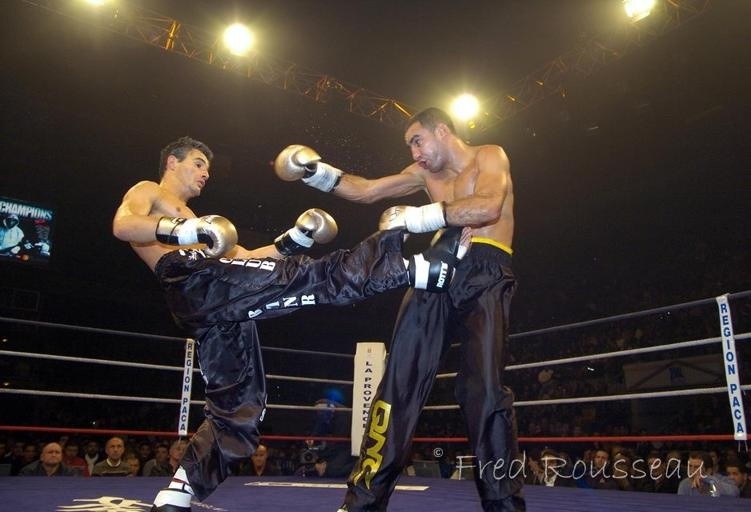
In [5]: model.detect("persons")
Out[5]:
[2,261,751,497]
[276,108,527,512]
[111,137,472,512]
[1,214,24,250]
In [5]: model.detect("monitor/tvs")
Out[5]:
[0,197,58,272]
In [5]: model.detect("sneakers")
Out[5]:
[408,224,474,291]
[150,488,212,511]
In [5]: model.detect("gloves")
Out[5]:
[155,215,237,257]
[275,145,342,194]
[379,200,447,235]
[275,207,338,257]
